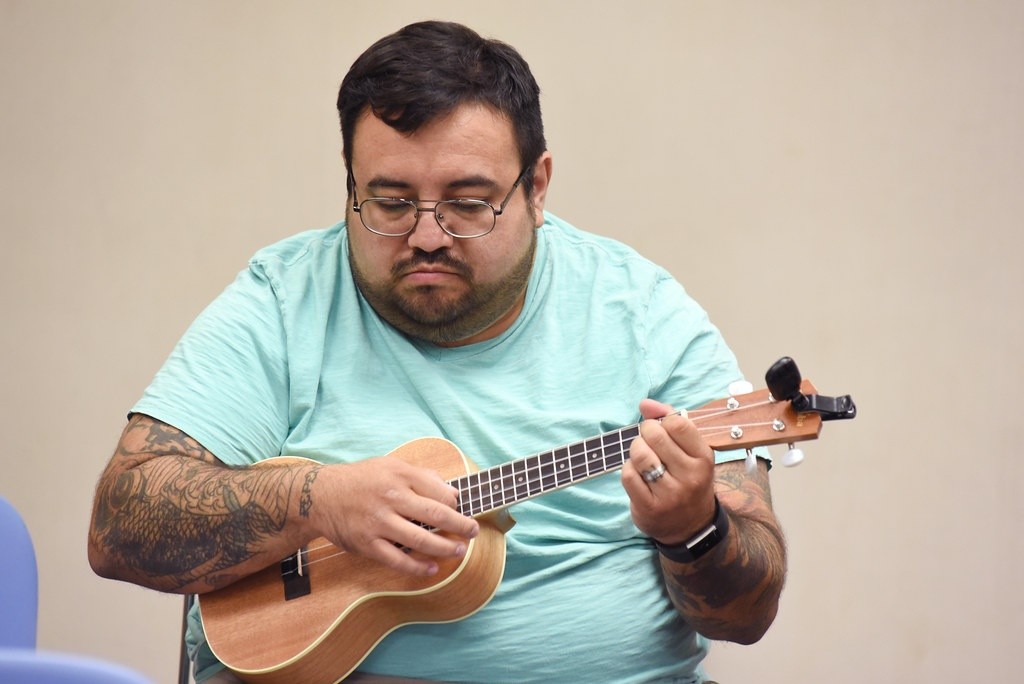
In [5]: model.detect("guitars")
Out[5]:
[197,355,857,684]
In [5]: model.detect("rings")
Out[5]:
[644,464,666,482]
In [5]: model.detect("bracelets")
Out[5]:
[652,494,730,563]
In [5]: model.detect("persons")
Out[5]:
[87,20,788,684]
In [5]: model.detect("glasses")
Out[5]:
[349,161,534,236]
